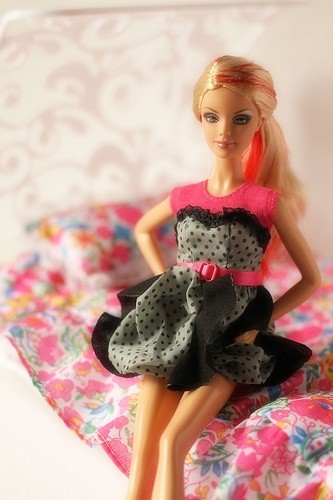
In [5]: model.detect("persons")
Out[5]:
[91,56,322,499]
